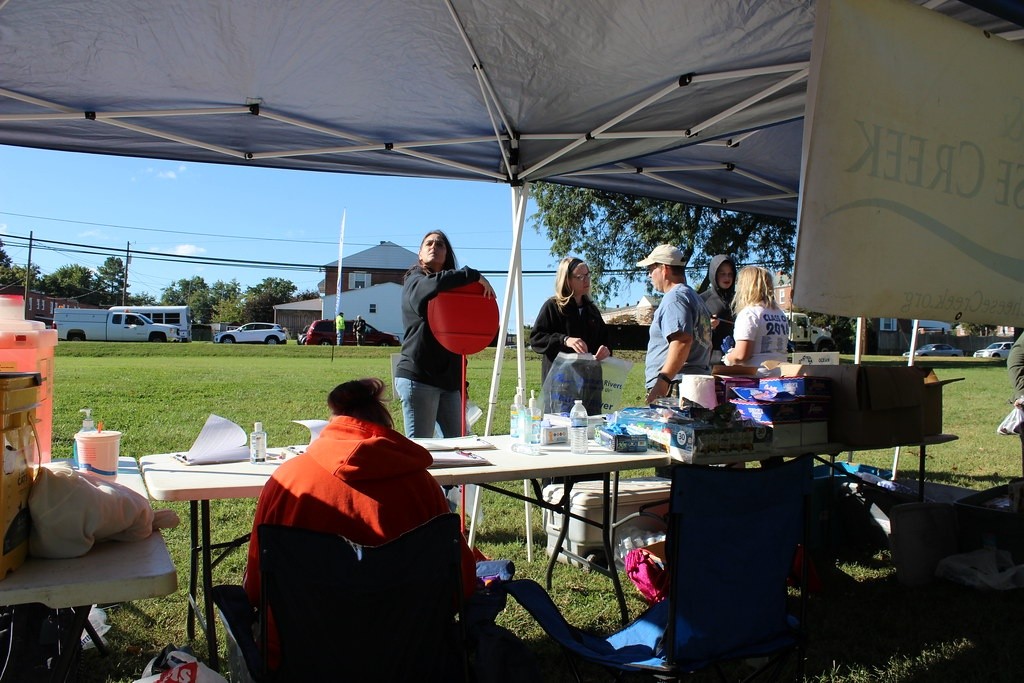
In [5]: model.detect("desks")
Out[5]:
[663,420,957,501]
[0,454,179,683]
[137,434,674,672]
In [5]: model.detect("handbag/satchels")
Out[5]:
[625,548,670,606]
[132,643,229,683]
[996,398,1024,435]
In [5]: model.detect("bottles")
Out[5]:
[569,400,589,455]
[249,422,267,465]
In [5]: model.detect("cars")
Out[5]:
[973,342,1015,358]
[902,344,963,357]
[297,325,310,345]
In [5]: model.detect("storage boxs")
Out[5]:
[538,474,672,571]
[595,354,963,458]
[952,481,1023,568]
[495,452,817,683]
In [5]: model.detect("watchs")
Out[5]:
[657,373,671,386]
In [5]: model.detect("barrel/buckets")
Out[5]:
[73,430,121,483]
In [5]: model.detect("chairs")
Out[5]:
[216,512,505,682]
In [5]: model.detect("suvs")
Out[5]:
[213,322,287,345]
[784,313,837,352]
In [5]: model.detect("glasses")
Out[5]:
[569,271,591,282]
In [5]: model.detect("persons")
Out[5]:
[699,255,737,364]
[635,244,713,479]
[353,315,366,346]
[394,230,497,515]
[336,312,345,347]
[1007,330,1024,477]
[244,379,486,676]
[529,256,613,486]
[727,266,789,378]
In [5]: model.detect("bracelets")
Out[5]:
[563,336,571,345]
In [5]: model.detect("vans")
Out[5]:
[108,305,191,339]
[305,319,402,346]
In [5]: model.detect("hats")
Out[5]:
[635,245,687,267]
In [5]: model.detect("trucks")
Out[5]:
[53,308,182,343]
